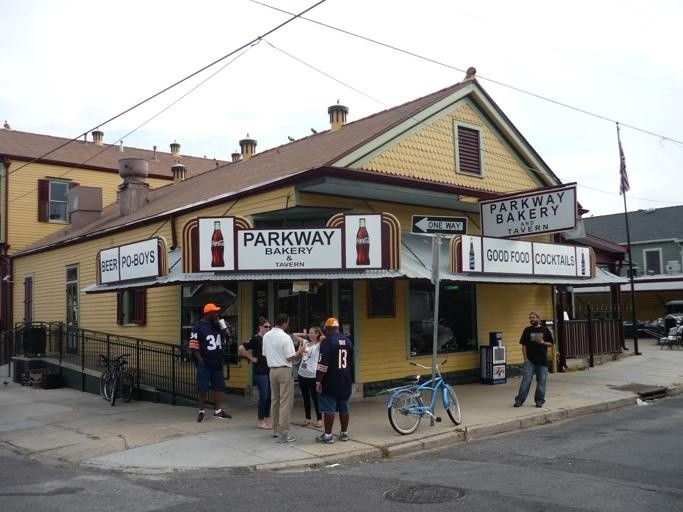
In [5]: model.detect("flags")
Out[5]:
[617,126,631,195]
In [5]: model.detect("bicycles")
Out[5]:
[98,352,134,406]
[381,360,462,434]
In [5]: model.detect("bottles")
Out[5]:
[356,218,369,265]
[211,220,224,267]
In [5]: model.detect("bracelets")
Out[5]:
[316,382,320,384]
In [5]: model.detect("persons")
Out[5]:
[261,312,306,442]
[238,317,272,429]
[189,303,232,423]
[315,317,354,443]
[514,312,553,407]
[292,326,327,427]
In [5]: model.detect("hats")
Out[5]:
[323,318,339,330]
[204,303,221,313]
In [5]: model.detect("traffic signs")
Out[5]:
[410,214,467,237]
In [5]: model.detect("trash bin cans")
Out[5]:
[24,326,47,358]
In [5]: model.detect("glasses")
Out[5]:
[264,326,271,330]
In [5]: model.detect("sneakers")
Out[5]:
[339,431,348,441]
[316,420,323,427]
[536,404,542,408]
[258,419,273,429]
[272,431,296,443]
[315,432,334,444]
[302,419,311,426]
[197,412,206,424]
[514,403,522,407]
[212,410,232,420]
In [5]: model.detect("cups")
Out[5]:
[218,319,227,329]
